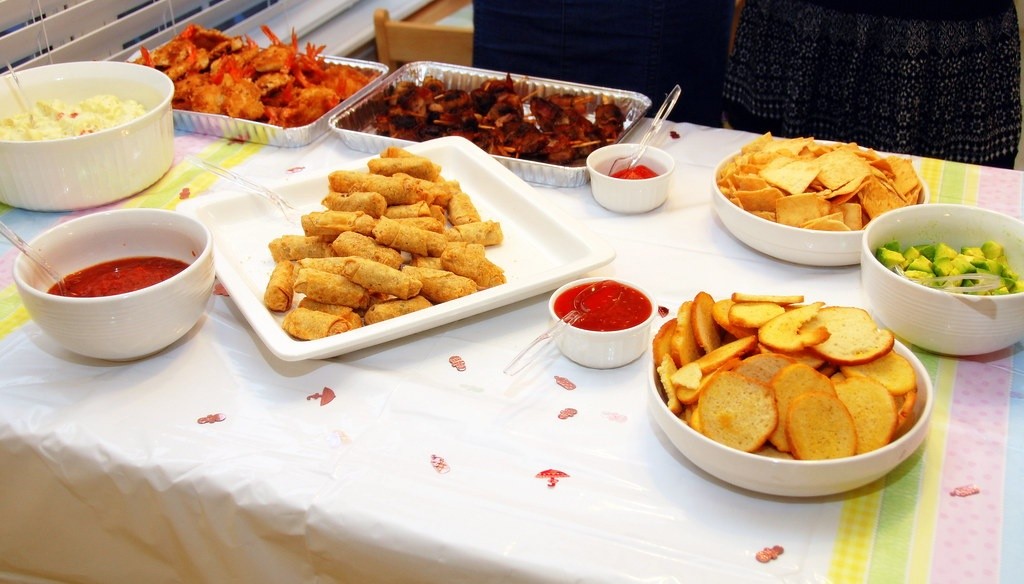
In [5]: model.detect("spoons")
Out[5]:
[609,84,681,179]
[502,280,621,377]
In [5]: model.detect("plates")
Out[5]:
[180,135,616,363]
[648,327,933,495]
[714,151,929,267]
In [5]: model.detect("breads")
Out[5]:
[651,291,919,460]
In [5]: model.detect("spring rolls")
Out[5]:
[264,146,509,340]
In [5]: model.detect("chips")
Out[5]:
[716,131,923,231]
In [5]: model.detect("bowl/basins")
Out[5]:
[547,275,658,369]
[587,143,674,214]
[0,59,173,213]
[861,205,1024,358]
[16,209,214,364]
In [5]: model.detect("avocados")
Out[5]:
[875,240,1024,296]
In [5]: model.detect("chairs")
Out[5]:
[372,8,474,78]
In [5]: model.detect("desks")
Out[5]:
[0,124,1024,584]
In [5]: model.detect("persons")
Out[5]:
[471,1,1023,169]
